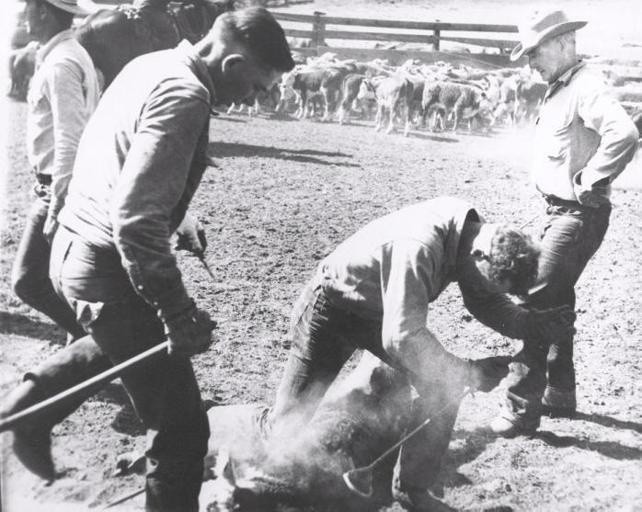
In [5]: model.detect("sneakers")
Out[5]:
[487,410,524,434]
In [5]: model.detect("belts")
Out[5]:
[36,174,53,185]
[543,195,590,208]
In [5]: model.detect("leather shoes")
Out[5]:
[2,377,60,482]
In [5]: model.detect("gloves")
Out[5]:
[174,215,207,257]
[465,355,512,393]
[526,305,574,348]
[165,305,211,357]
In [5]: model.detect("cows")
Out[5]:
[228,51,549,138]
[195,347,420,511]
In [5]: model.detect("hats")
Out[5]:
[510,9,587,62]
[47,0,90,15]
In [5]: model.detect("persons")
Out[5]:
[199,194,575,512]
[482,9,640,438]
[7,0,99,342]
[0,5,295,512]
